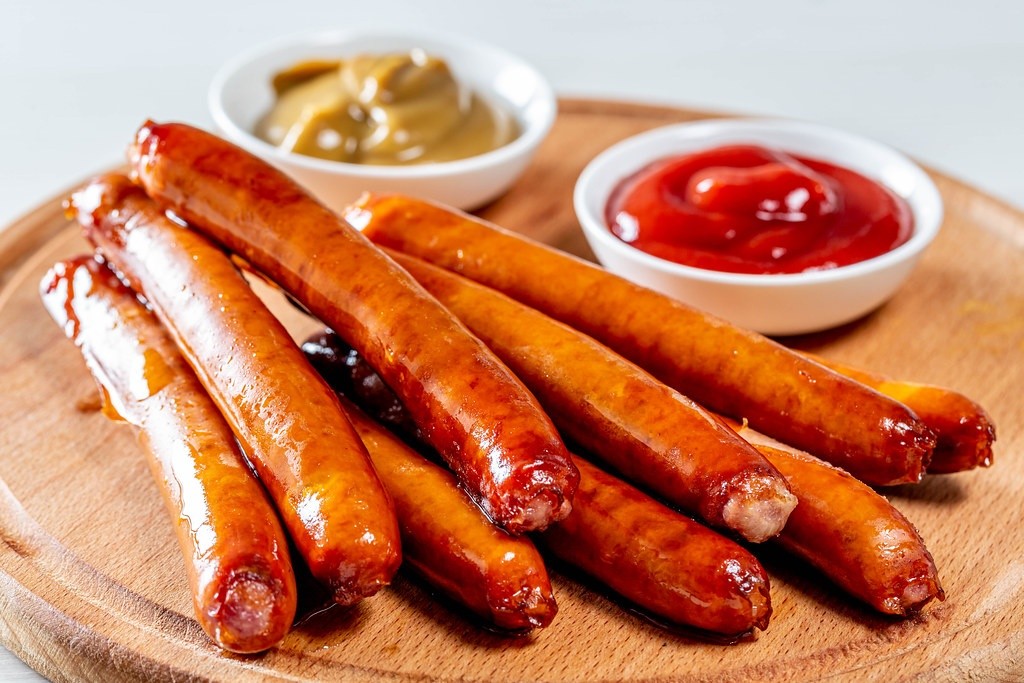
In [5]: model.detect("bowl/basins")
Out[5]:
[575,117,943,337]
[206,20,560,218]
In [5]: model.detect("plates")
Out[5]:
[0,96,1023,682]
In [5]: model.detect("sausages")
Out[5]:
[39,119,996,656]
